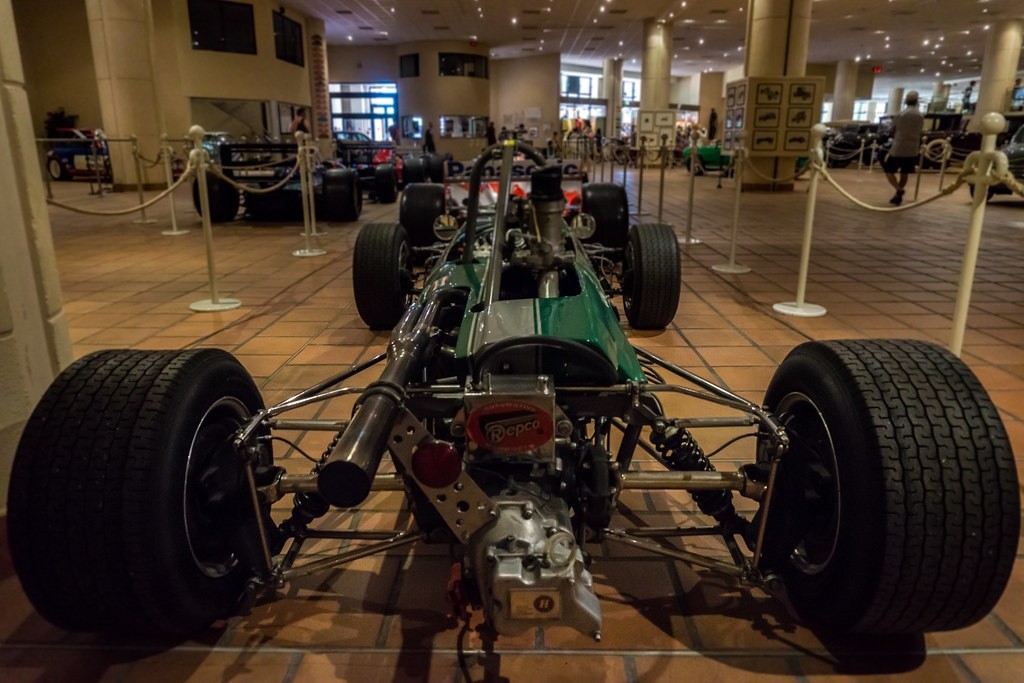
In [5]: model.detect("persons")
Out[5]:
[881,90,925,205]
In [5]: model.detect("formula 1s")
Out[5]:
[4,132,1022,660]
[36,102,1022,260]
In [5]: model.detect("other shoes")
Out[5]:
[891,189,904,205]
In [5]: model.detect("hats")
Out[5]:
[907,91,918,100]
